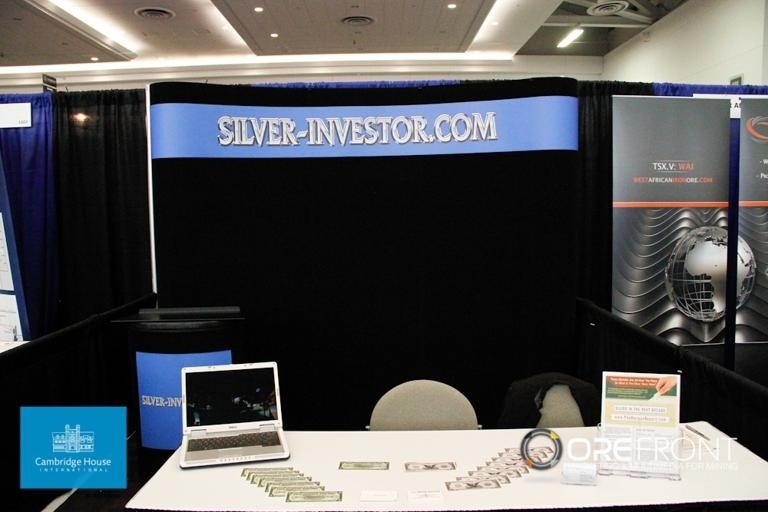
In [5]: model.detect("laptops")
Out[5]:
[180,361,290,469]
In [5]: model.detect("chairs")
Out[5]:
[490,372,601,428]
[364,375,483,432]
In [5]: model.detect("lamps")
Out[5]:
[554,21,587,51]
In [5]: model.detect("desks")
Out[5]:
[120,415,768,512]
